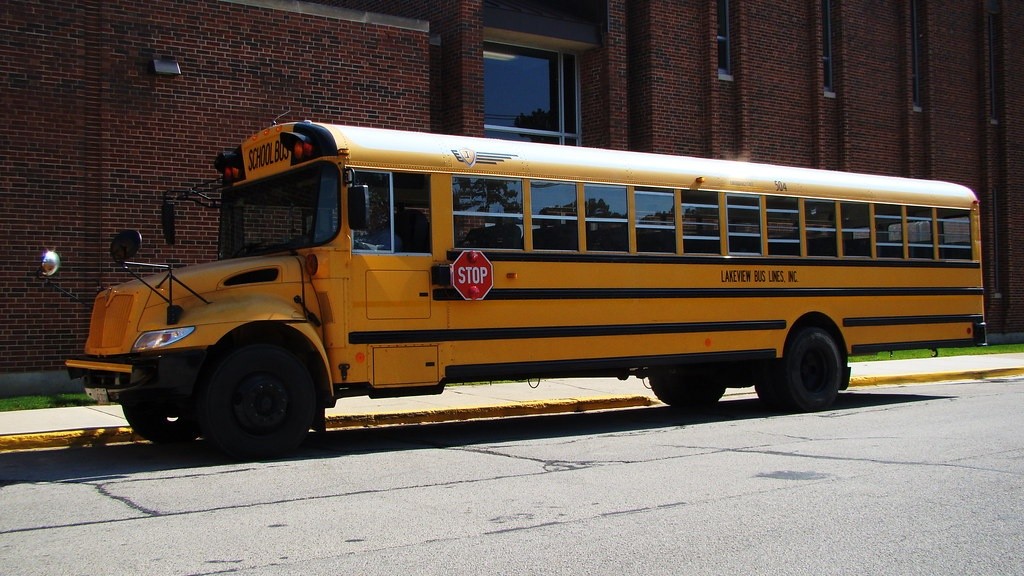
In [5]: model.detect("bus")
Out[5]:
[45,120,988,464]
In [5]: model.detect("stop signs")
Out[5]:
[449,250,494,301]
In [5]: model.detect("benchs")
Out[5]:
[378,208,974,260]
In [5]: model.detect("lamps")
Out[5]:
[152,55,181,76]
